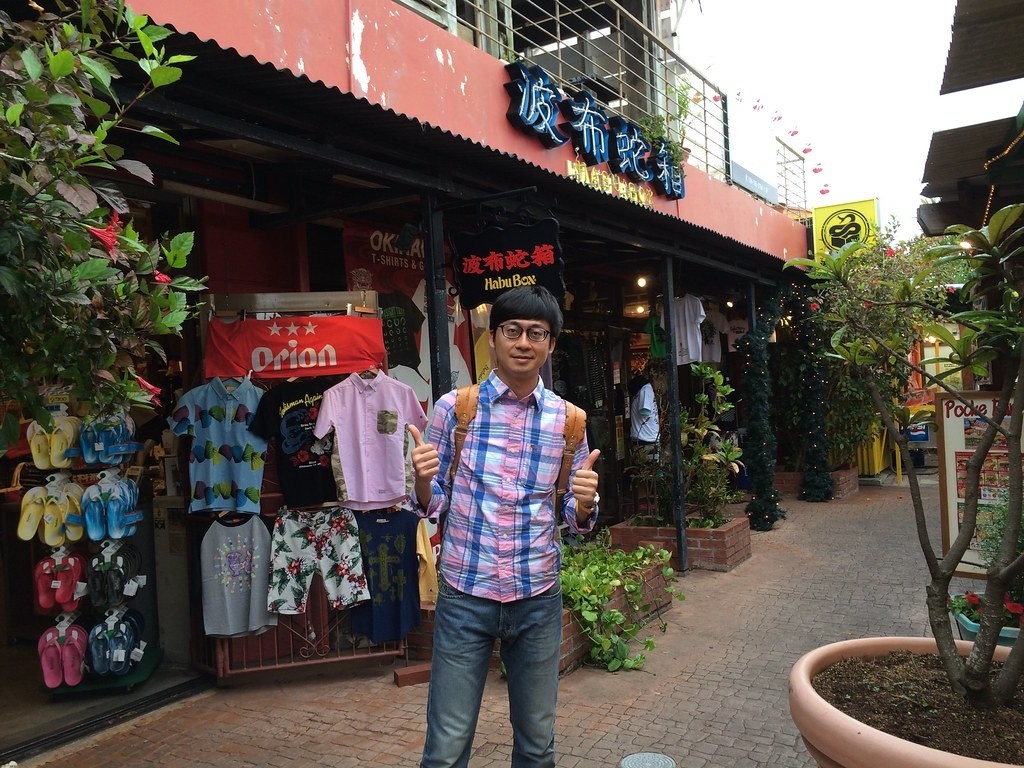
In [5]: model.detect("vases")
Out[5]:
[950,592,1020,646]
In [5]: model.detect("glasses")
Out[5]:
[496,324,551,342]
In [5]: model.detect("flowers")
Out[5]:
[945,589,1023,629]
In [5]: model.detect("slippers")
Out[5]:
[16,414,144,688]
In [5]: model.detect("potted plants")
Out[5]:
[783,203,1024,768]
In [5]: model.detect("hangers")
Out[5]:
[33,403,127,646]
[655,283,745,320]
[208,363,402,520]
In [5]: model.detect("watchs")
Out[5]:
[577,493,600,514]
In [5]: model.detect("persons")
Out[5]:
[408,287,600,768]
[629,376,660,482]
